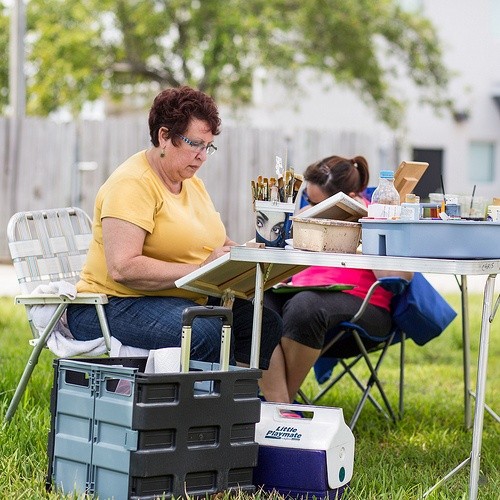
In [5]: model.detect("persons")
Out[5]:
[256,211,285,242]
[249,155,414,404]
[65,86,283,370]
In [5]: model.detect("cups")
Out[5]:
[254,200,296,250]
[428,192,500,222]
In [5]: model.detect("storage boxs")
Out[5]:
[358,216,500,259]
[46,355,264,500]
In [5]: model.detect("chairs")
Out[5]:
[300,187,458,431]
[3,207,150,422]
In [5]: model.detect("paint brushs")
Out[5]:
[261,183,265,201]
[264,178,268,201]
[268,178,277,201]
[285,172,291,203]
[289,166,294,187]
[278,177,285,203]
[256,176,261,200]
[250,181,257,200]
[440,175,446,201]
[292,178,303,203]
[469,185,476,217]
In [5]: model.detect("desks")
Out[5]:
[230,245,500,500]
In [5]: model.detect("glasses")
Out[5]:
[175,132,217,156]
[305,197,319,207]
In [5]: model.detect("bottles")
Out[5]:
[404,193,424,219]
[371,170,400,206]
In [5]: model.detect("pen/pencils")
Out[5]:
[202,245,214,253]
